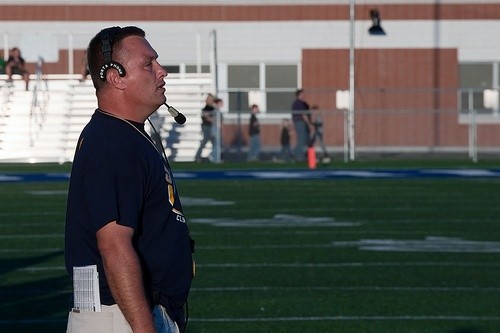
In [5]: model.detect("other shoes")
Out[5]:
[208,155,217,164]
[195,155,203,163]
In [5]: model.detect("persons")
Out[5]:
[192,86,228,165]
[246,105,262,163]
[270,89,333,165]
[0,47,30,91]
[64,26,196,333]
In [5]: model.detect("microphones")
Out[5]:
[163,101,186,125]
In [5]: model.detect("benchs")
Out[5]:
[0,73,216,160]
